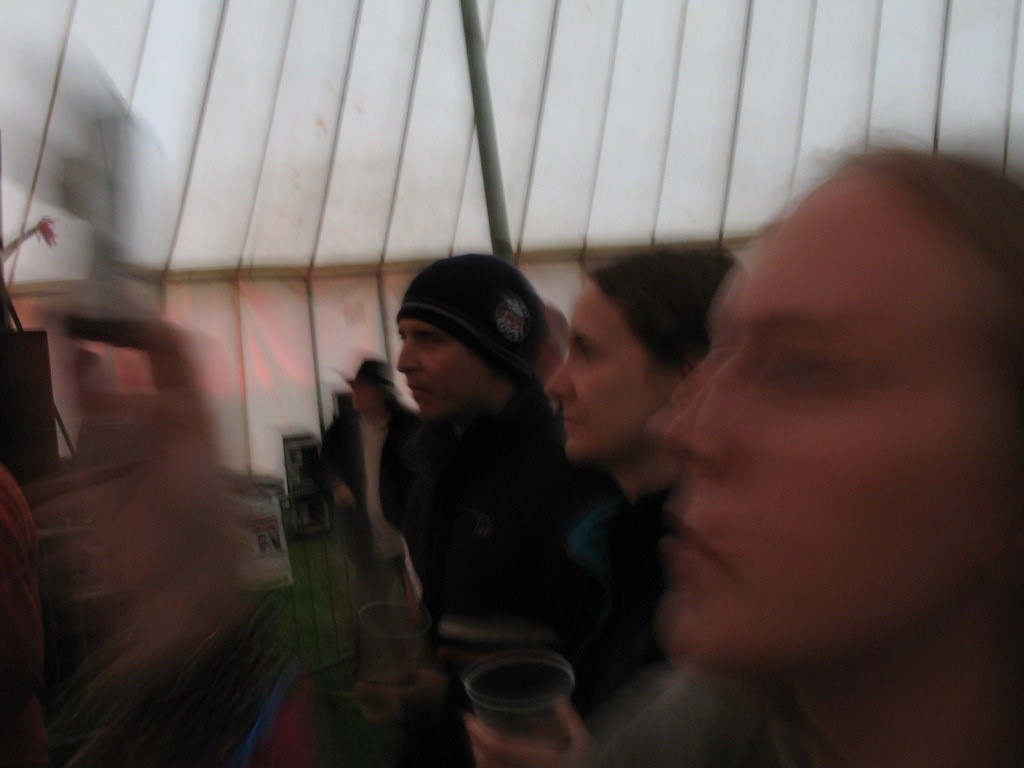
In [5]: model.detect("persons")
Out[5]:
[0,129,1024,768]
[378,254,618,699]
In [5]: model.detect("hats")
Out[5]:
[394,253,543,390]
[588,241,736,369]
[350,359,397,391]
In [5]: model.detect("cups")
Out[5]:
[356,601,432,687]
[461,648,573,750]
